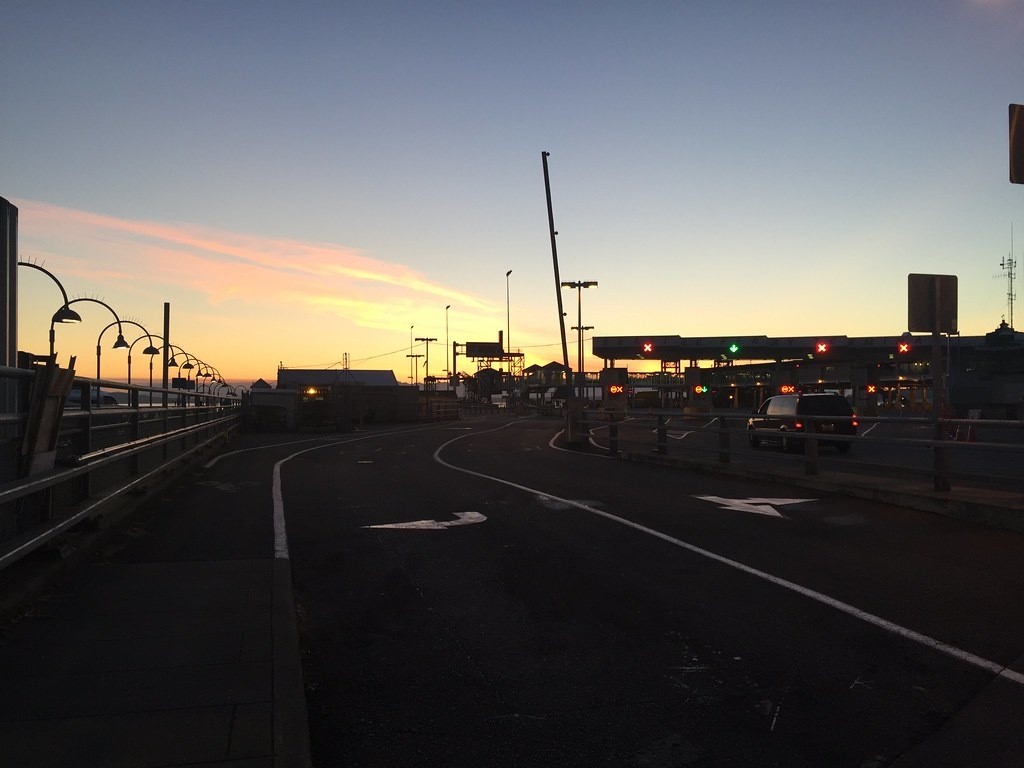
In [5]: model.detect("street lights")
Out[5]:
[446,305,450,390]
[506,270,512,372]
[410,325,415,385]
[18,262,249,406]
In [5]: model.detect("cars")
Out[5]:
[747,392,858,455]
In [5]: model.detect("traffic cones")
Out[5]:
[955,417,976,442]
